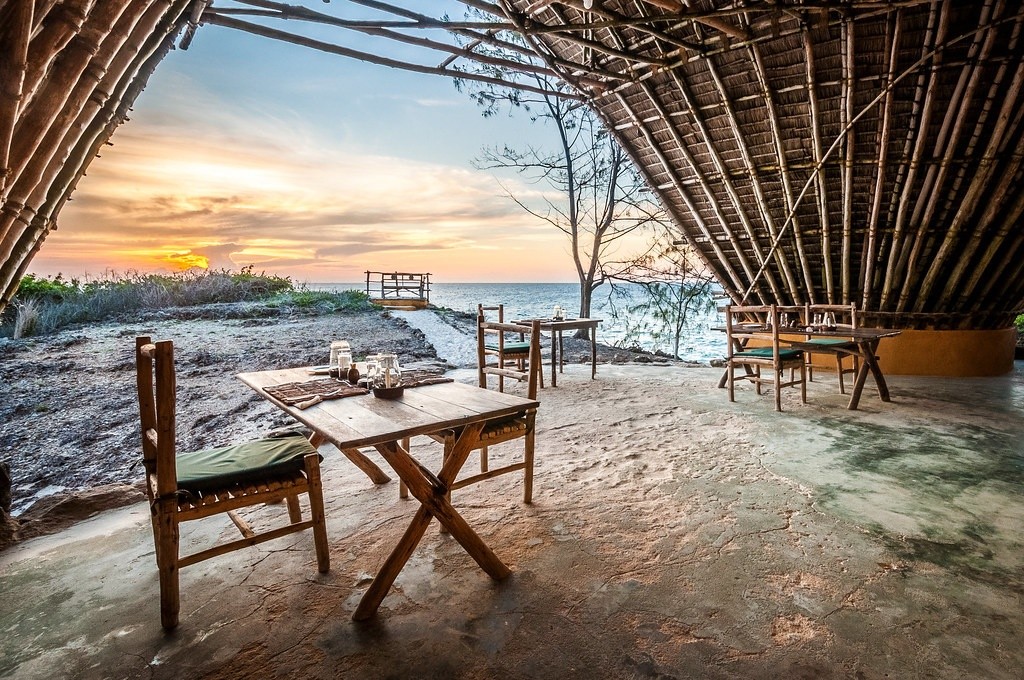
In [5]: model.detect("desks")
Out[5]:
[236,361,540,621]
[511,318,604,386]
[711,323,903,411]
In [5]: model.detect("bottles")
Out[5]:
[813,314,822,333]
[328,341,352,381]
[552,305,567,322]
[366,351,402,392]
[780,313,788,329]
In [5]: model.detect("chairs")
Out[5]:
[399,314,540,533]
[724,305,806,412]
[478,303,544,393]
[804,302,859,394]
[136,336,330,627]
